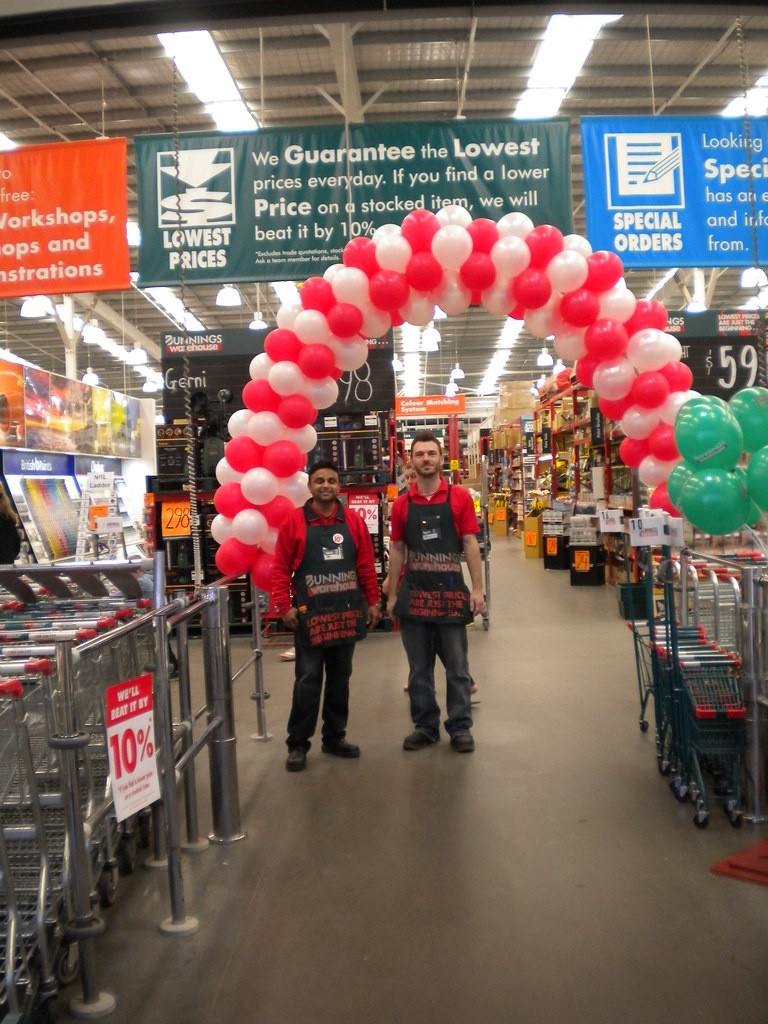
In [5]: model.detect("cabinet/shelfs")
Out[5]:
[480,379,649,588]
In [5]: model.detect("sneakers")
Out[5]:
[402,730,441,749]
[450,727,475,752]
[320,737,361,757]
[286,748,307,772]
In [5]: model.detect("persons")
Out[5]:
[273,460,381,772]
[382,433,484,752]
[0,481,21,564]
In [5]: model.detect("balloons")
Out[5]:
[212,205,768,576]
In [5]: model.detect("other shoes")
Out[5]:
[171,659,182,678]
[403,682,437,696]
[278,646,296,661]
[470,684,481,704]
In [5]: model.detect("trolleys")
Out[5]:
[0,599,157,1024]
[620,543,768,659]
[625,619,749,829]
[462,505,492,632]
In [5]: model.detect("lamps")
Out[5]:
[20,13,768,401]
